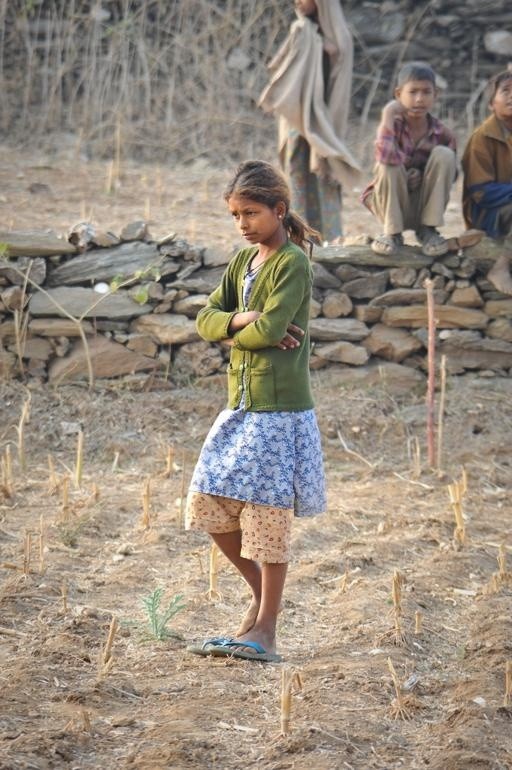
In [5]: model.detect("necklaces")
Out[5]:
[243,256,266,287]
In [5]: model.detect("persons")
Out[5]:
[185,159,327,660]
[257,0,364,245]
[359,62,458,256]
[460,71,512,239]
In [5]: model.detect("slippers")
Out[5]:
[187,636,283,662]
[371,227,450,257]
